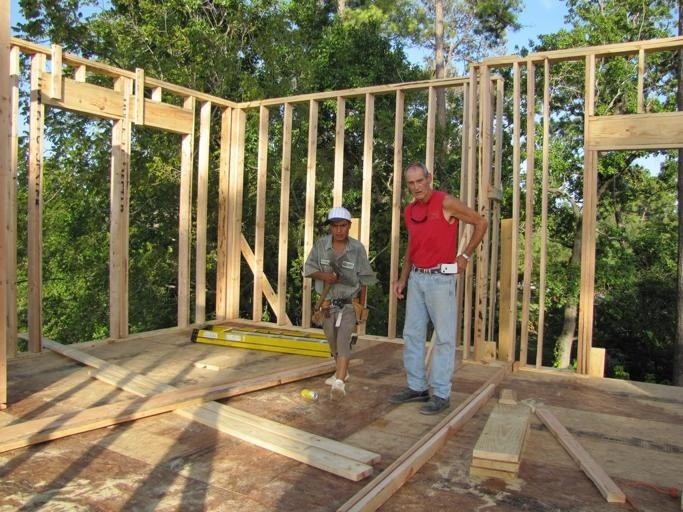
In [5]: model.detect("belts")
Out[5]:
[415,268,442,274]
[323,299,353,309]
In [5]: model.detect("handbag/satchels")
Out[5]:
[312,307,324,326]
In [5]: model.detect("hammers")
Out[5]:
[314,261,345,311]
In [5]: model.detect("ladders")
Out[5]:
[191,325,357,358]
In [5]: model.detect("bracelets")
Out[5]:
[462,254,470,262]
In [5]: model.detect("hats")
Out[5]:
[324,207,352,225]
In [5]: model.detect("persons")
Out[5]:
[305,207,375,402]
[387,163,488,415]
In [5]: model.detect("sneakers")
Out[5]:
[326,369,350,401]
[388,388,430,403]
[420,395,449,415]
